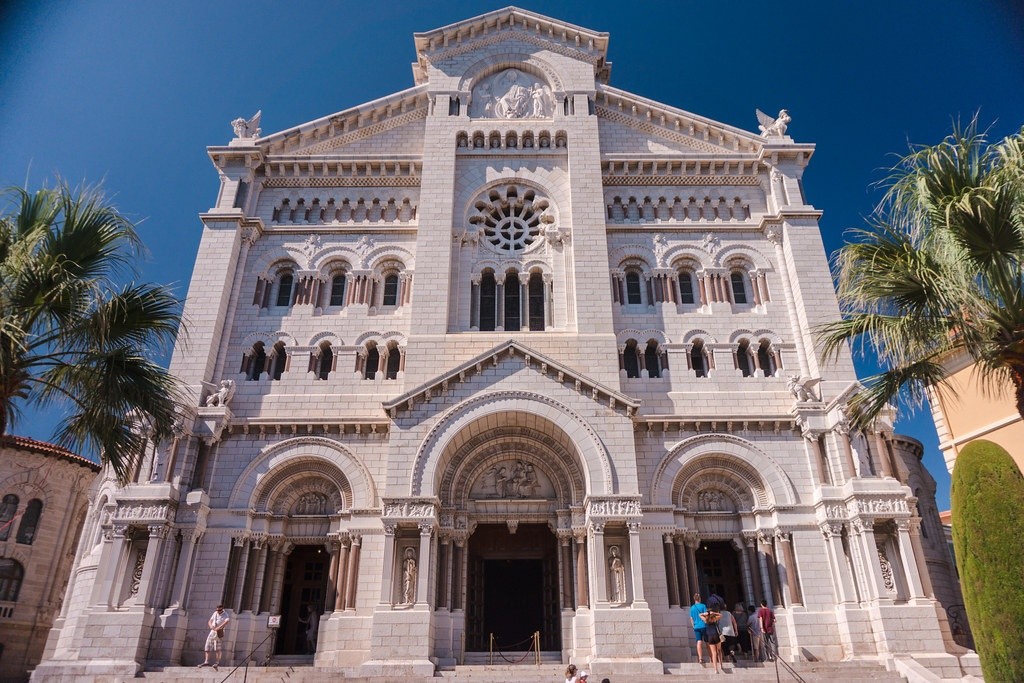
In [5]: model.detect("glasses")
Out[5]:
[215,609,222,612]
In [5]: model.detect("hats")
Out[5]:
[579,671,589,678]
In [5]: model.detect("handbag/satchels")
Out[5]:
[719,634,726,643]
[216,630,223,638]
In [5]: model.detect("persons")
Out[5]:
[478,83,493,118]
[607,546,624,602]
[698,491,726,511]
[487,461,537,499]
[690,593,776,673]
[655,234,661,254]
[403,548,417,604]
[848,422,873,478]
[302,235,320,255]
[532,83,547,119]
[299,493,326,515]
[299,605,318,652]
[198,604,230,668]
[356,237,373,254]
[500,71,528,118]
[703,233,716,253]
[565,665,610,683]
